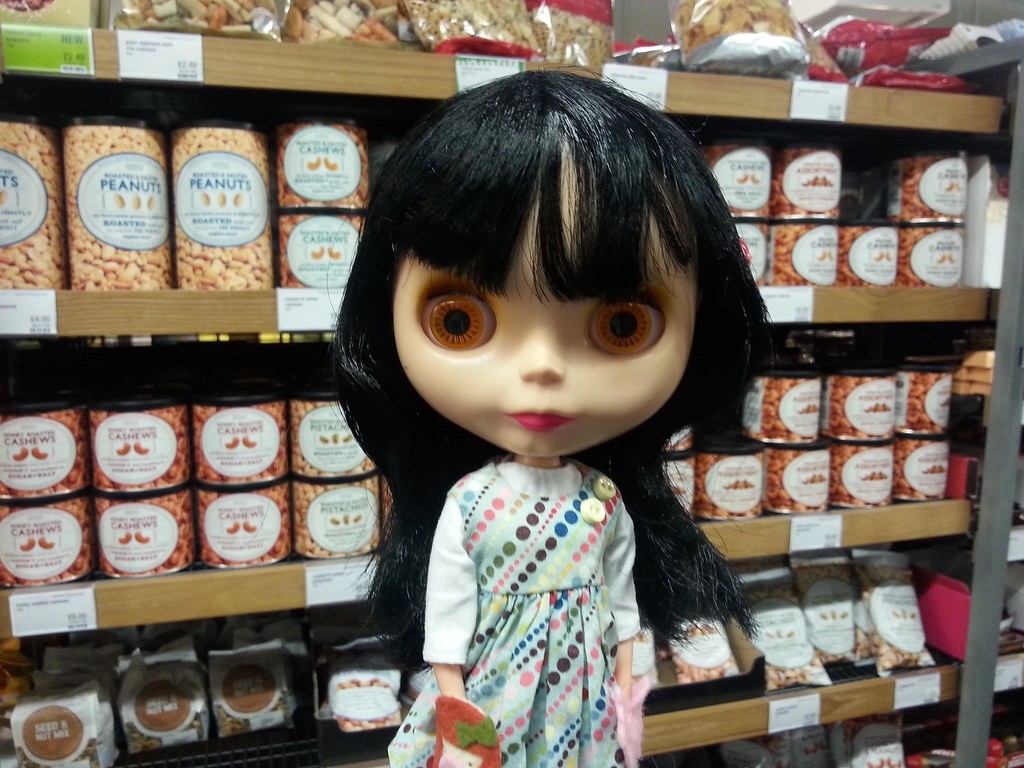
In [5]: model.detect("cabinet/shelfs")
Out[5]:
[0,21,1024,768]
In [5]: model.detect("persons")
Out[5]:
[338,73,773,767]
